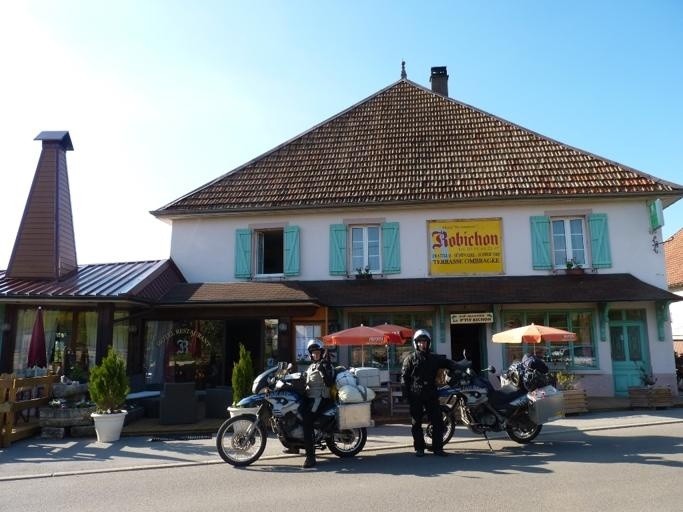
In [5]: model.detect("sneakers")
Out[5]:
[414,448,449,457]
[283,449,316,468]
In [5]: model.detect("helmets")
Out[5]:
[411,328,432,341]
[306,338,325,351]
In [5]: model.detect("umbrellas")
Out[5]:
[321,323,391,365]
[27,306,47,368]
[372,321,414,385]
[491,323,578,353]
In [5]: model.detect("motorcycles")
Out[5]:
[422,351,565,450]
[217,358,380,465]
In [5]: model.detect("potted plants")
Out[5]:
[553,372,589,419]
[627,360,675,411]
[224,342,259,437]
[87,346,130,443]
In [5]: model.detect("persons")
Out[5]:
[400,328,474,457]
[281,339,336,466]
[523,347,549,374]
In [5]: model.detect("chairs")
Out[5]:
[125,373,233,425]
